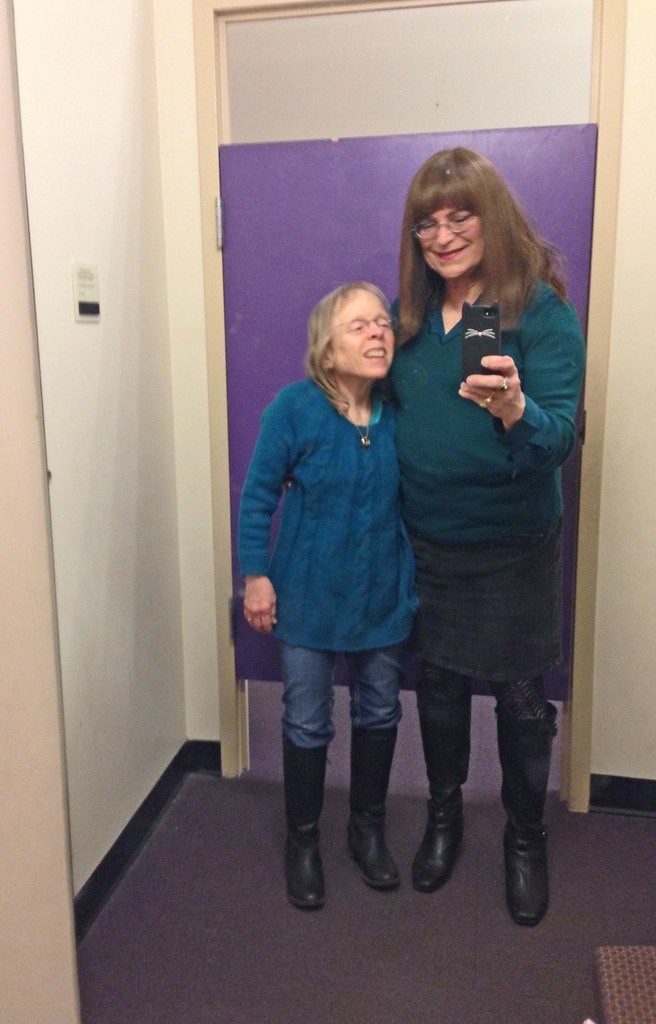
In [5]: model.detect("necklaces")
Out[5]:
[349,401,372,447]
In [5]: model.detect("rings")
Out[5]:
[486,390,494,404]
[480,400,489,408]
[499,377,508,390]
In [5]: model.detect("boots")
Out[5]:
[412,675,472,893]
[493,700,558,926]
[282,731,325,907]
[346,725,400,886]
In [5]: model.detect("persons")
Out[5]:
[388,149,586,925]
[237,282,419,909]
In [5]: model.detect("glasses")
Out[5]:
[410,211,476,238]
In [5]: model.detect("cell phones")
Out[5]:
[462,301,501,382]
